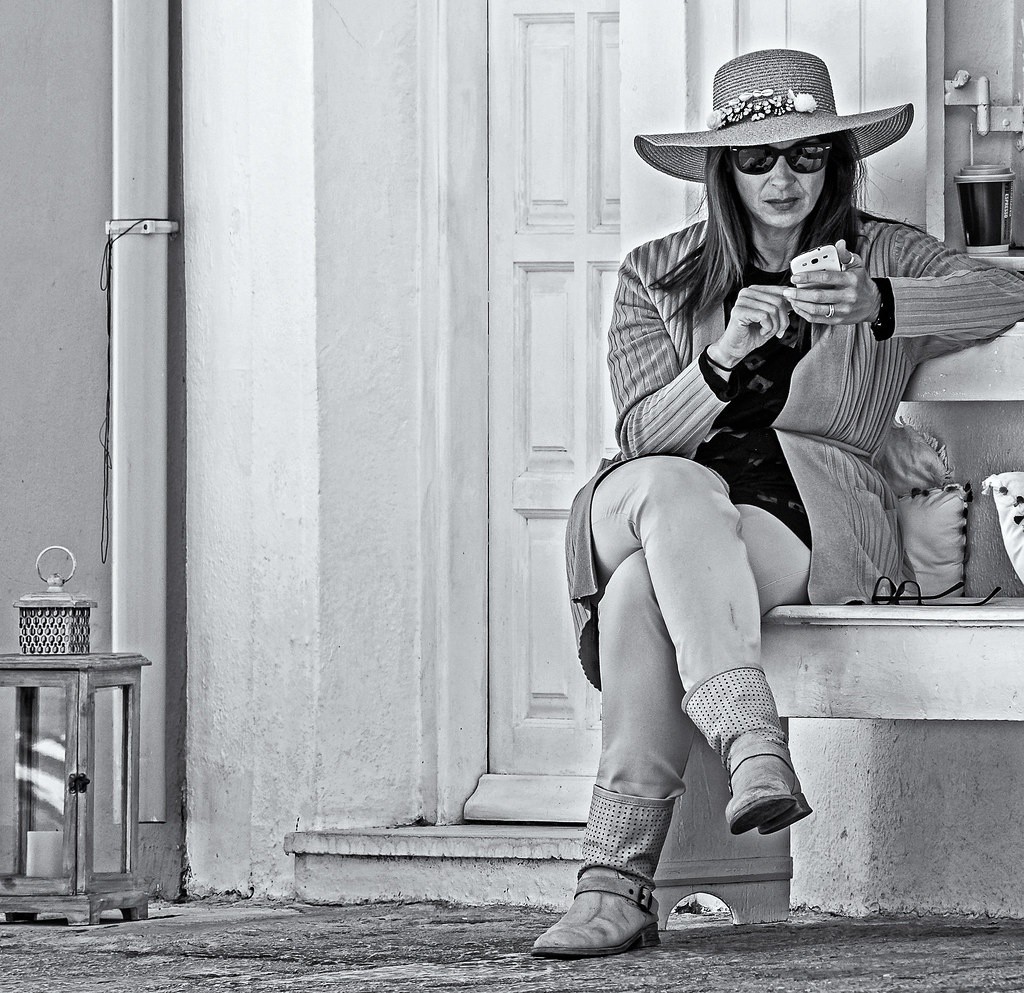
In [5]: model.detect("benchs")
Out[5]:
[761,595,1024,723]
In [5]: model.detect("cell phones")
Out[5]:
[790,245,842,292]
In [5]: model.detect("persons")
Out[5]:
[528,48,1024,959]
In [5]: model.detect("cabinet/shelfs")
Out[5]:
[0,652,152,927]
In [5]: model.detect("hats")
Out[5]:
[633,49,915,184]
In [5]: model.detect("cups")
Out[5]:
[953,164,1017,255]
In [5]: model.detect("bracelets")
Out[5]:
[705,344,734,371]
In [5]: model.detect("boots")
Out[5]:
[680,662,813,835]
[531,784,675,956]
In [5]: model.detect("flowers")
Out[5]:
[793,93,818,115]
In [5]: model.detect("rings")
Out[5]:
[825,302,835,318]
[841,251,855,266]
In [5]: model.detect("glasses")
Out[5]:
[871,575,1002,606]
[729,139,833,176]
